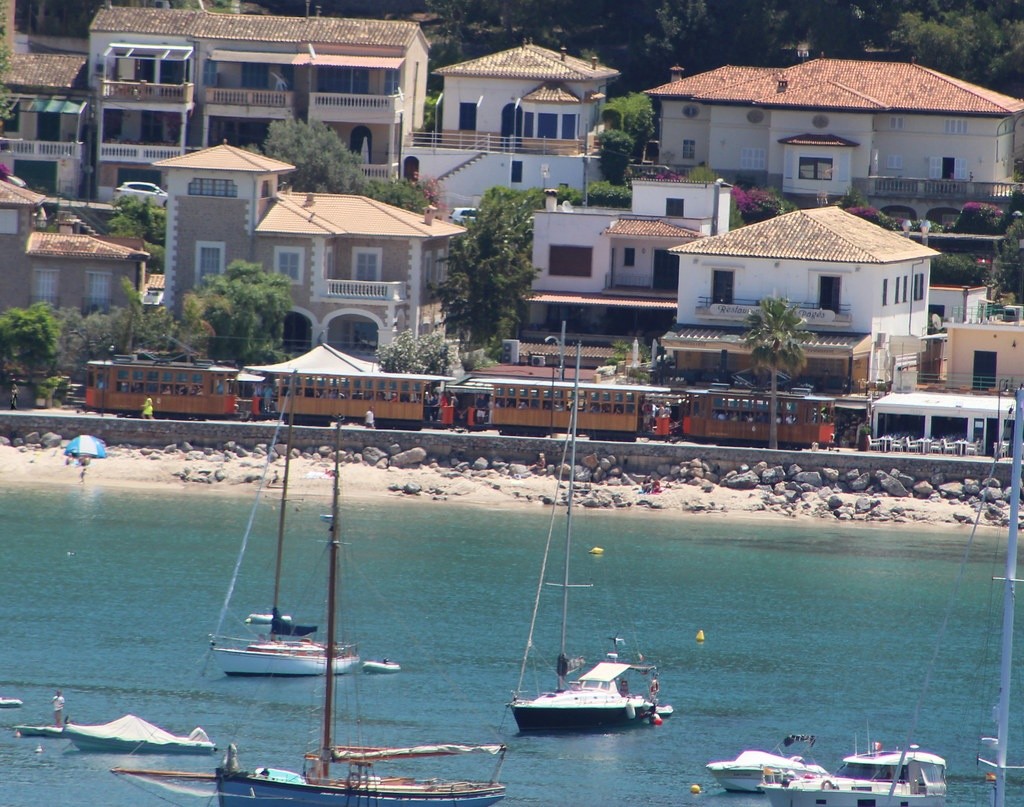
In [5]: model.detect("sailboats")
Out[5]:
[204,370,358,676]
[875,385,1024,807]
[505,340,662,732]
[109,412,511,807]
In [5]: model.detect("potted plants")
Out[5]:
[858,428,871,451]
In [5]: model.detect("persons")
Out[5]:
[620,681,629,697]
[268,470,280,486]
[140,393,155,420]
[530,451,546,470]
[251,382,274,412]
[10,383,20,409]
[425,387,494,424]
[51,690,64,729]
[834,413,873,452]
[714,407,830,425]
[637,471,662,493]
[325,468,339,478]
[496,399,623,415]
[296,387,417,403]
[219,380,233,394]
[118,382,204,395]
[65,451,89,483]
[649,678,659,702]
[641,394,672,437]
[365,405,375,428]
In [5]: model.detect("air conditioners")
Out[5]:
[531,355,545,367]
[502,339,520,364]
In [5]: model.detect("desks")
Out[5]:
[878,438,894,452]
[954,440,967,456]
[917,439,932,455]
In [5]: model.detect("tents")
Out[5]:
[64,434,107,459]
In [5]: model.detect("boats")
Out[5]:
[705,735,830,795]
[756,722,950,807]
[11,723,66,740]
[66,715,217,756]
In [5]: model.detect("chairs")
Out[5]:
[867,434,984,457]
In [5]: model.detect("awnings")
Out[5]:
[5,95,89,141]
